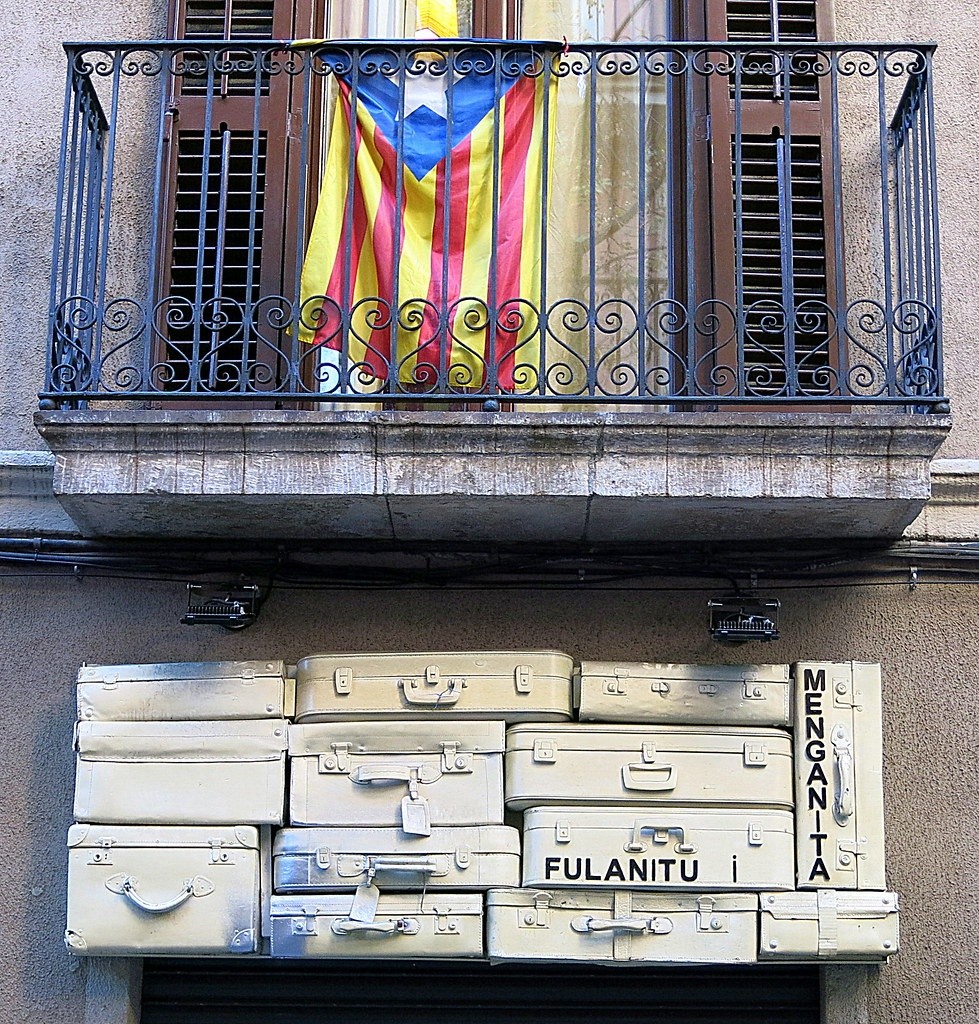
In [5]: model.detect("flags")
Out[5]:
[287,34,559,390]
